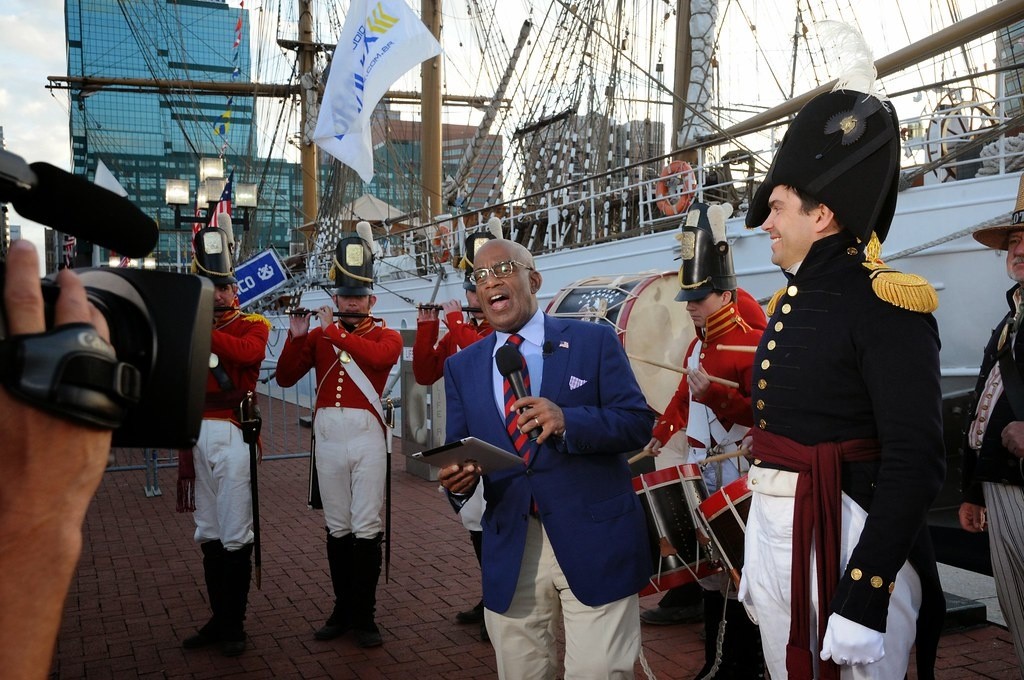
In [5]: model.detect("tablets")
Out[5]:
[412,436,526,476]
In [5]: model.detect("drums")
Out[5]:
[631,463,726,598]
[694,470,753,587]
[544,268,698,418]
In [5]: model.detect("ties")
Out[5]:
[502,334,538,514]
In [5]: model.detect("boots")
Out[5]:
[181,540,252,656]
[455,530,490,642]
[314,528,383,648]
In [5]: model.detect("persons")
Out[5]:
[413,233,495,643]
[636,202,770,680]
[437,237,653,680]
[0,240,116,680]
[735,90,950,680]
[958,173,1023,667]
[172,227,270,658]
[277,238,406,648]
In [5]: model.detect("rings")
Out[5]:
[534,418,540,426]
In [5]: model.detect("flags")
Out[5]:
[61,231,78,266]
[313,0,444,183]
[191,1,245,256]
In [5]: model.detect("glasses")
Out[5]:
[469,260,533,285]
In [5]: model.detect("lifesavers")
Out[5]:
[655,161,697,216]
[434,225,449,262]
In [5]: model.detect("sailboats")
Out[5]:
[42,1,1024,405]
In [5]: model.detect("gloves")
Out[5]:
[819,613,885,666]
[737,566,759,625]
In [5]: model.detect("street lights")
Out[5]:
[164,156,257,229]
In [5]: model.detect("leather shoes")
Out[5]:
[640,605,705,627]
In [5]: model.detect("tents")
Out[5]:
[292,194,420,258]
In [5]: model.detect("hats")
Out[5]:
[745,89,901,245]
[673,203,739,303]
[333,237,375,296]
[194,228,239,286]
[463,232,498,291]
[973,173,1024,251]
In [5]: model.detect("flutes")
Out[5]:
[213,306,240,311]
[415,303,483,313]
[283,308,374,318]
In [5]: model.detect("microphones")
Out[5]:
[543,341,553,353]
[495,345,542,442]
[11,161,159,259]
